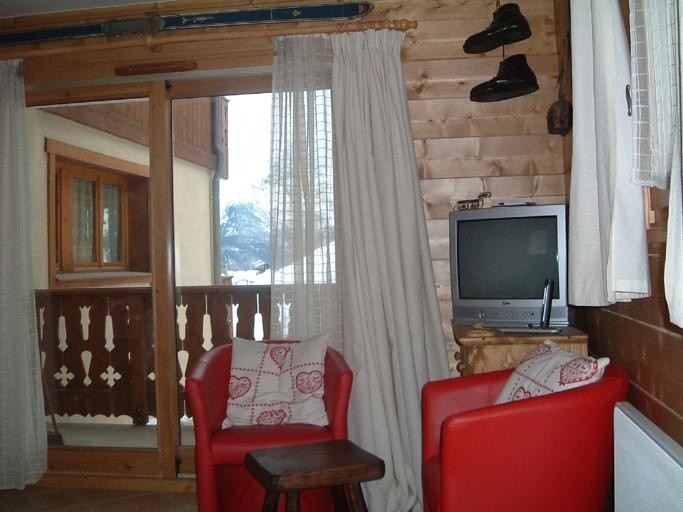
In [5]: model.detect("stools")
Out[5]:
[241,434,387,512]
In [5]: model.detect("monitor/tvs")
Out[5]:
[448,205,571,329]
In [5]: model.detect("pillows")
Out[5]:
[221,337,331,429]
[490,337,612,410]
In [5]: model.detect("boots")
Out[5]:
[463,3,531,55]
[469,53,539,102]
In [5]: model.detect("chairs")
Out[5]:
[418,357,631,510]
[183,336,357,511]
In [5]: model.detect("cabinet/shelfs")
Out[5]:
[448,319,590,375]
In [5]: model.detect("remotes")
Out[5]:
[539,277,554,328]
